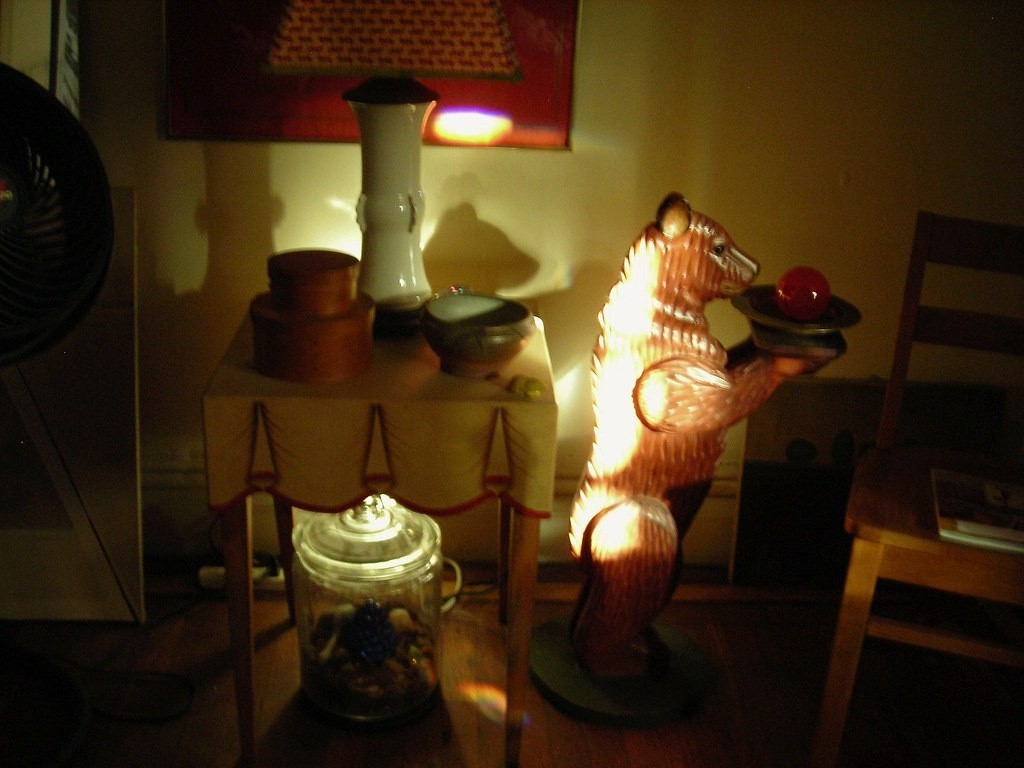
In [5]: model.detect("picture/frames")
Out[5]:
[162,0,580,152]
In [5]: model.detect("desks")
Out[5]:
[201,292,559,768]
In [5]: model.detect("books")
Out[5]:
[930,463,1024,553]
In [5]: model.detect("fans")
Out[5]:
[0,60,117,366]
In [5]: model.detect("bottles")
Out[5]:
[293,490,459,720]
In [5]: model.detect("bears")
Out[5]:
[566,189,842,680]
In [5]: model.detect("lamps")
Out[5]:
[263,0,525,342]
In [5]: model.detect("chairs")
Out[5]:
[807,208,1024,768]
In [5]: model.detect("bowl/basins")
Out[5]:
[421,289,535,379]
[730,283,862,356]
